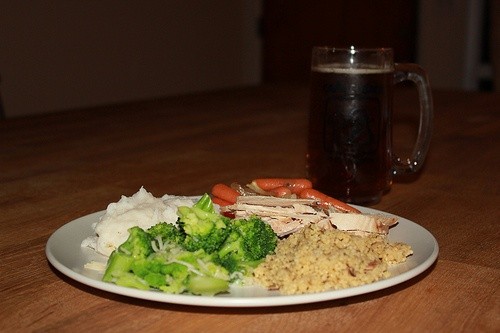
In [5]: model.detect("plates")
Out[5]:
[45,196,440,308]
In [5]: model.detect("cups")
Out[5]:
[307,44,433,206]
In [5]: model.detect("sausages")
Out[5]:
[211,176,361,216]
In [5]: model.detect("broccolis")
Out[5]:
[100,194,278,295]
